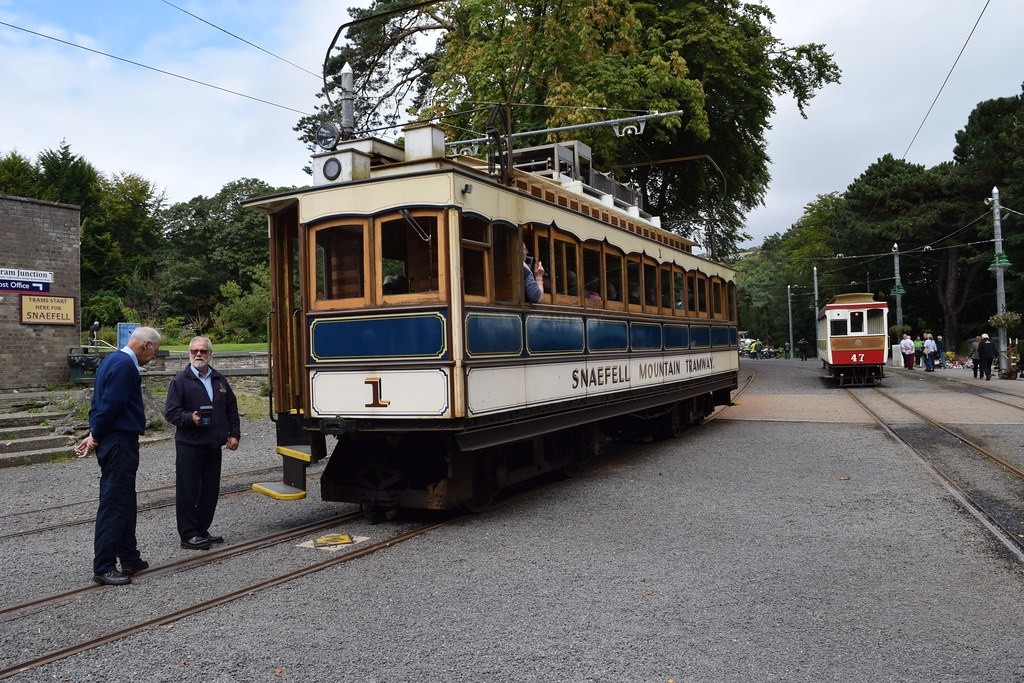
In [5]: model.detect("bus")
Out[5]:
[237,124,740,526]
[816,291,891,386]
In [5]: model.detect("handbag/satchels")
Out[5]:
[923,348,930,353]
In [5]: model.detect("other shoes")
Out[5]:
[974,374,977,377]
[924,368,934,372]
[986,377,990,380]
[908,367,915,370]
[980,374,983,377]
[989,375,991,378]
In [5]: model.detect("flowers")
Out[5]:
[945,351,957,361]
[956,355,967,365]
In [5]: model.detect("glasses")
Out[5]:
[190,349,209,355]
[150,343,159,355]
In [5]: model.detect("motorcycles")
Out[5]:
[739,346,783,360]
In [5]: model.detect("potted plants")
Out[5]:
[889,324,912,337]
[987,311,1022,330]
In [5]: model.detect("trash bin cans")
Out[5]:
[70,355,97,379]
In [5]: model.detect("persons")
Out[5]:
[88,322,99,346]
[741,339,764,359]
[73,327,161,585]
[384,275,407,295]
[784,339,791,360]
[165,336,241,550]
[798,337,808,361]
[969,334,1024,380]
[900,332,947,372]
[523,242,669,308]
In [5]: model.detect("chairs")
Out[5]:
[383,275,402,294]
[393,274,410,293]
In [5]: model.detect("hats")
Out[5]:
[981,334,989,339]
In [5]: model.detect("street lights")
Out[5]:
[987,251,1008,373]
[889,283,906,343]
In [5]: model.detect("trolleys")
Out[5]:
[998,337,1018,379]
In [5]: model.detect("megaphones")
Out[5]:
[892,247,897,252]
[984,197,994,205]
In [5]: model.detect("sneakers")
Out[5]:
[93,569,131,585]
[121,558,148,575]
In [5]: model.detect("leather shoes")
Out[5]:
[205,534,224,543]
[181,535,211,549]
